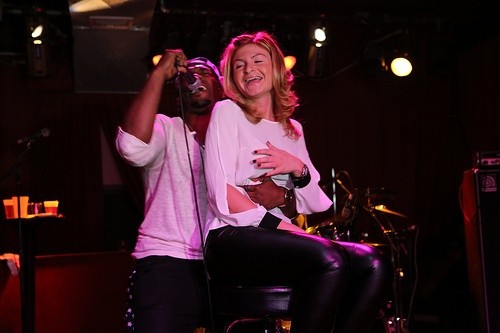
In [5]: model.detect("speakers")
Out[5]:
[461,169,500,333]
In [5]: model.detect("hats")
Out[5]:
[177,57,224,93]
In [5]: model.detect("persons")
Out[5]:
[117,49,298,333]
[203,30,391,333]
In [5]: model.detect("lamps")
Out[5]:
[378,50,412,78]
[26,5,44,37]
[313,16,339,42]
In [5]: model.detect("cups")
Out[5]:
[29,202,43,215]
[43,200,60,215]
[3,199,15,219]
[12,196,29,218]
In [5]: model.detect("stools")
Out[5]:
[223,284,291,333]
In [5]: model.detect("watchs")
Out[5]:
[293,165,308,181]
[278,190,292,207]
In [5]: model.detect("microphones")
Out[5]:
[17,128,51,144]
[176,54,201,91]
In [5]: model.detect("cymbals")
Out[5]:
[336,178,385,192]
[369,205,407,218]
